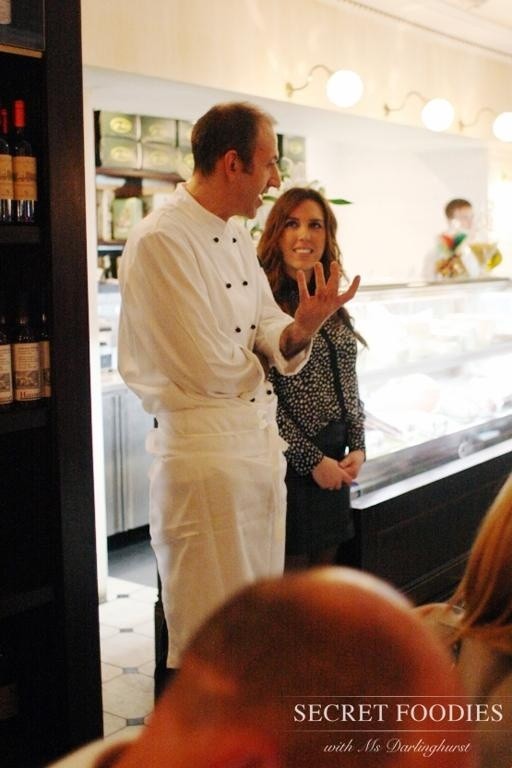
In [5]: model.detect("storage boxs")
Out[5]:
[94,110,305,186]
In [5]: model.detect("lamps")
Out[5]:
[286,64,359,106]
[385,92,459,133]
[461,110,512,142]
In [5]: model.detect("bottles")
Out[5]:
[0,96,56,407]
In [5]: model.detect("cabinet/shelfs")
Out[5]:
[98,386,155,537]
[95,167,184,279]
[0,0,103,768]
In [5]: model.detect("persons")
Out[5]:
[256,188,366,572]
[50,565,480,768]
[409,472,512,768]
[423,199,493,281]
[118,102,361,710]
